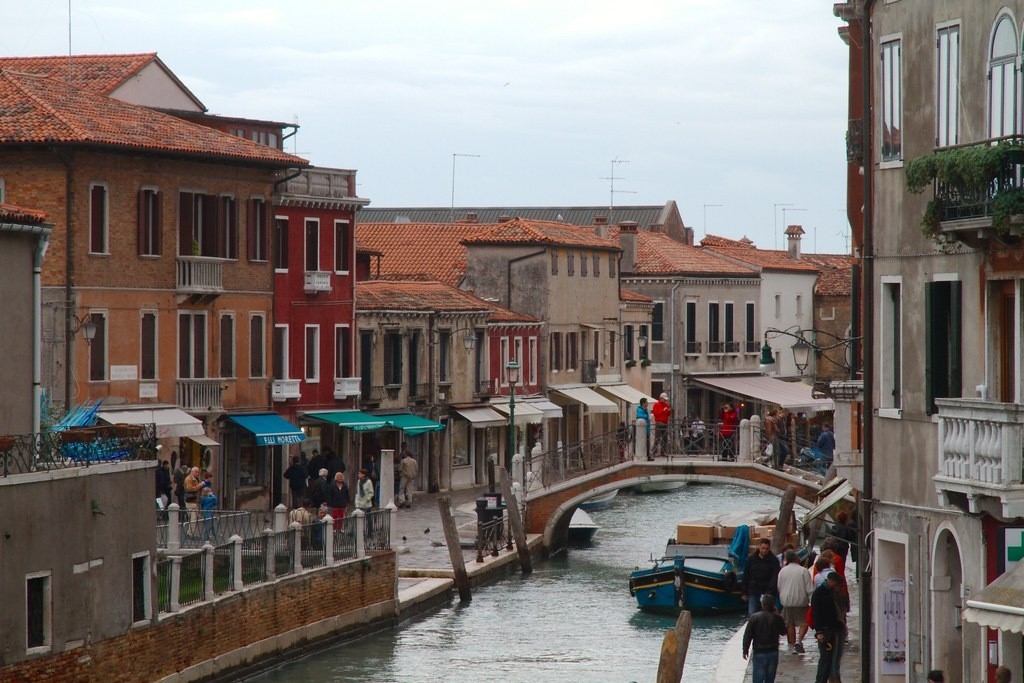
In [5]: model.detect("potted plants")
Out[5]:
[641,359,653,368]
[626,360,636,369]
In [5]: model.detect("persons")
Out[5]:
[762,408,837,477]
[742,509,863,655]
[617,393,740,462]
[152,459,218,541]
[811,572,849,683]
[283,443,419,549]
[743,593,787,683]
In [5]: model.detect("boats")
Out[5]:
[579,489,620,509]
[626,544,811,614]
[568,507,600,541]
[633,481,689,492]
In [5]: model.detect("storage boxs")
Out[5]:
[678,510,799,552]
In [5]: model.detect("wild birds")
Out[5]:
[402,535,407,542]
[423,527,430,535]
[430,539,446,551]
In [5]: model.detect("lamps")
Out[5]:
[760,329,862,372]
[617,331,647,349]
[73,313,102,344]
[442,328,477,354]
[791,329,866,375]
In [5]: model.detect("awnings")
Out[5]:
[227,412,444,446]
[683,370,836,414]
[455,372,653,428]
[91,405,205,439]
[962,557,1024,638]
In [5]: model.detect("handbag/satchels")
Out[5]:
[765,443,773,455]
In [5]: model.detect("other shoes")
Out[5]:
[660,393,669,400]
[792,640,805,655]
[762,463,769,467]
[398,502,405,508]
[405,504,411,509]
